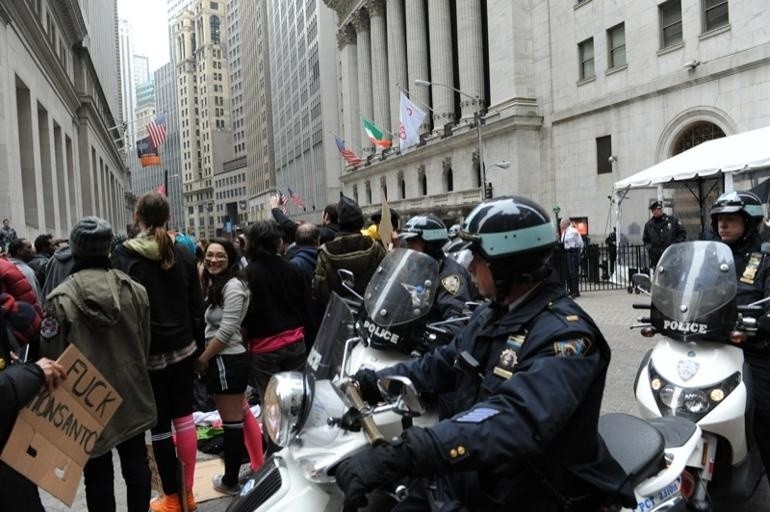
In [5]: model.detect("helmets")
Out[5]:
[710,190,765,241]
[445,195,557,302]
[393,214,449,254]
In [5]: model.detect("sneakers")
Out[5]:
[238,463,255,484]
[149,492,183,512]
[568,291,580,298]
[186,492,197,512]
[212,471,241,495]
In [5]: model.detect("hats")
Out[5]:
[371,208,399,220]
[336,191,362,224]
[70,216,112,259]
[649,201,662,210]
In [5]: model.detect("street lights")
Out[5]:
[162,169,180,231]
[553,206,561,238]
[413,78,511,205]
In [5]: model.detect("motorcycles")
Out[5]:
[334,248,483,393]
[628,241,766,512]
[221,293,704,512]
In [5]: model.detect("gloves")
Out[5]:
[351,368,386,404]
[326,412,443,507]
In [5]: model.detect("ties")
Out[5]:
[563,232,566,247]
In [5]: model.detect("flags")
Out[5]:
[136,114,167,168]
[398,93,426,156]
[362,118,391,149]
[334,138,361,169]
